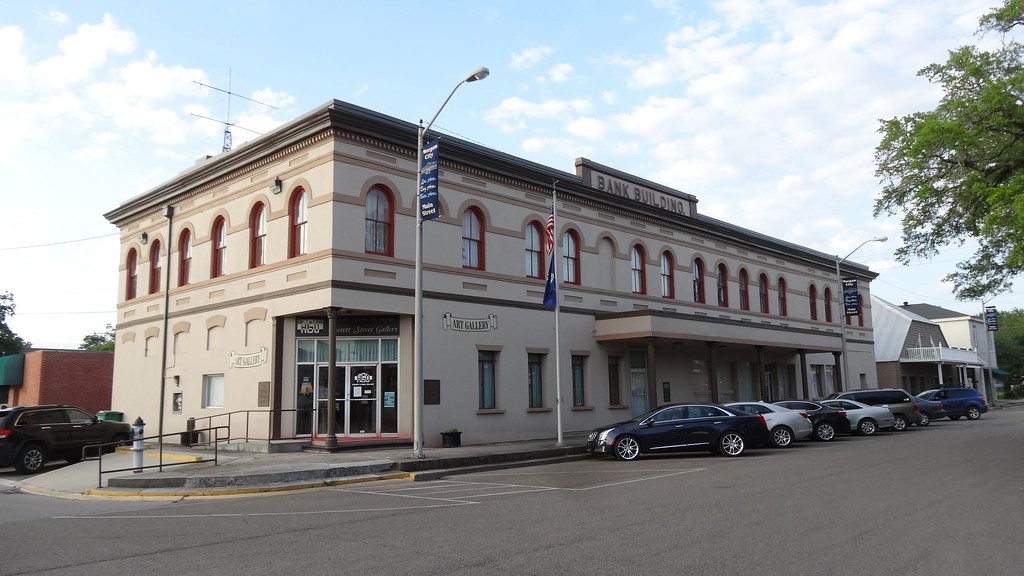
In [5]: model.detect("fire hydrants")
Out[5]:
[128,416,146,472]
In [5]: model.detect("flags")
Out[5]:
[545,202,554,256]
[543,251,556,310]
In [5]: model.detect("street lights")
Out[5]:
[414,66,490,459]
[982,287,1012,407]
[835,236,889,391]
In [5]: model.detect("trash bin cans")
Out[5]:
[96,410,123,422]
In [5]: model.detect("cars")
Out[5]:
[0,405,136,476]
[913,386,991,427]
[729,389,925,448]
[586,403,767,457]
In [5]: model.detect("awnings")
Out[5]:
[0,354,24,385]
[992,370,1012,377]
